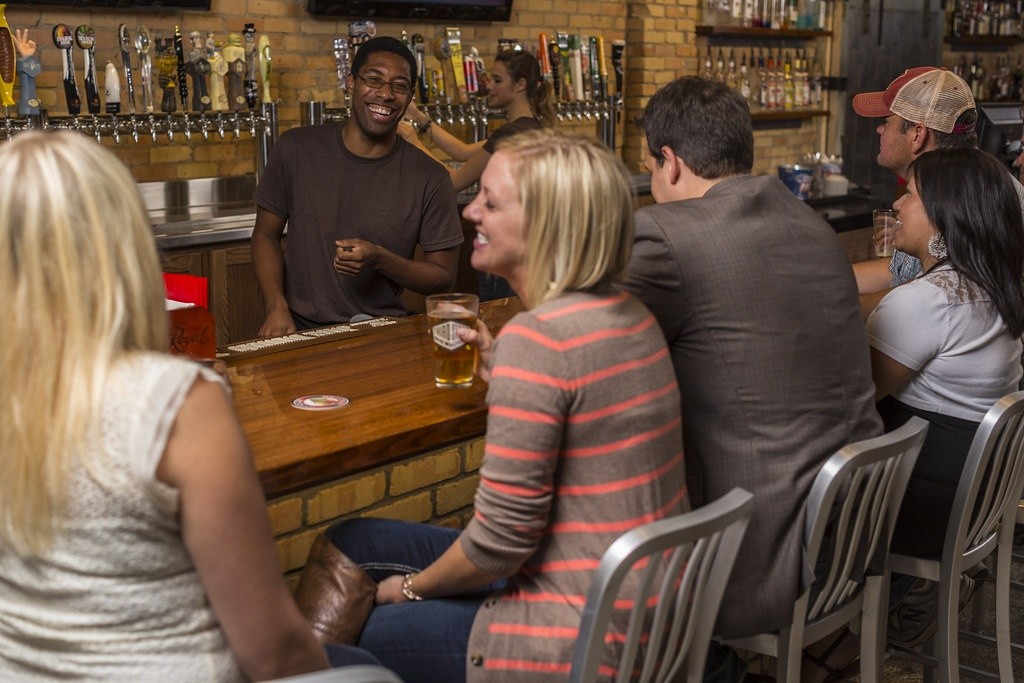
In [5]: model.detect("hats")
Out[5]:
[852,66,978,133]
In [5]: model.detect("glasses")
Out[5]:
[358,73,413,95]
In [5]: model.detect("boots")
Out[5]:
[292,533,377,645]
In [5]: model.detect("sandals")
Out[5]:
[802,627,860,683]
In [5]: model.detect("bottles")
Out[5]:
[696,0,1024,111]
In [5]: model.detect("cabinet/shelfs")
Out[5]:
[163,242,267,347]
[695,23,832,120]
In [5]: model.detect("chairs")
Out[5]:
[848,389,1024,683]
[710,416,930,683]
[570,486,757,683]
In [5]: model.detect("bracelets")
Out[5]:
[402,572,422,601]
[416,116,431,139]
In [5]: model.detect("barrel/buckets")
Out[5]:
[779,163,816,200]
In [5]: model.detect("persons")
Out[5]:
[848,64,1024,293]
[205,31,223,59]
[873,137,1024,250]
[622,76,884,640]
[12,27,36,56]
[0,130,384,683]
[227,33,241,45]
[800,147,1024,683]
[403,49,556,300]
[291,128,695,683]
[186,31,203,60]
[251,35,464,340]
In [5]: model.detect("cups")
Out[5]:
[873,209,898,260]
[425,293,478,389]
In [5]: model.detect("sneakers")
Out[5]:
[887,574,975,648]
[964,562,989,592]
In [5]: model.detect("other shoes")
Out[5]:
[702,646,748,683]
